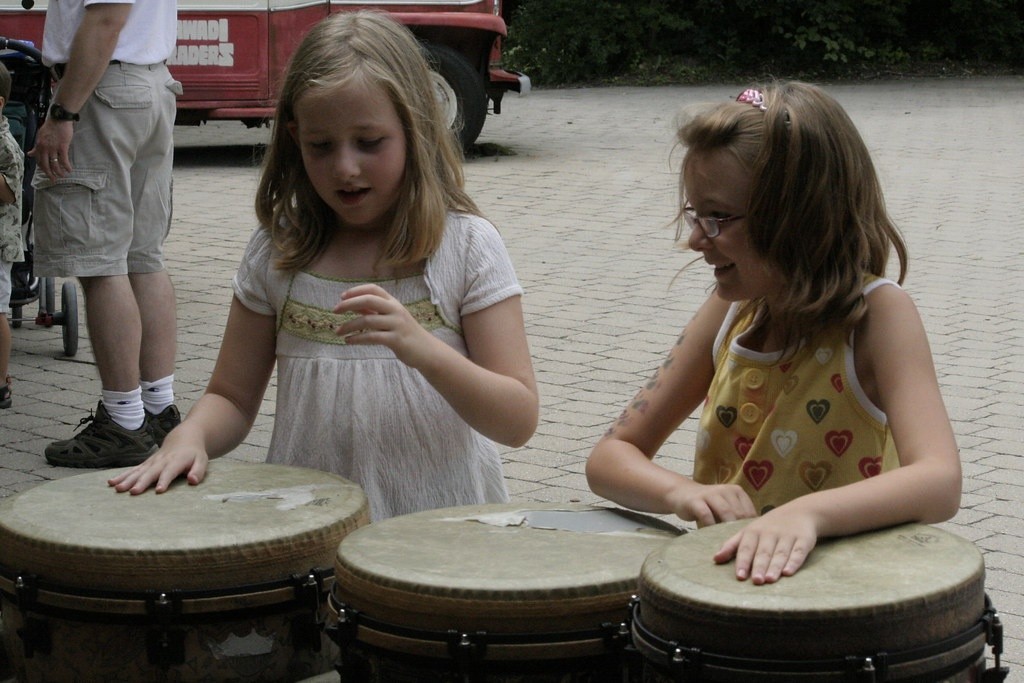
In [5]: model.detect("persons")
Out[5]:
[0,0,190,468]
[108,10,540,525]
[585,83,963,585]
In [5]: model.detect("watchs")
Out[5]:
[46,102,80,123]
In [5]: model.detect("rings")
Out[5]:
[49,158,58,162]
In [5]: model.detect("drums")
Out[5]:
[335,492,1009,683]
[1,453,373,683]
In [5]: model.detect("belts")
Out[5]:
[48,60,121,82]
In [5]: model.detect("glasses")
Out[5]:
[681,208,744,239]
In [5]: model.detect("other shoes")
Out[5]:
[0,375,13,409]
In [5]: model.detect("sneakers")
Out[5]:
[45,401,160,468]
[144,404,182,447]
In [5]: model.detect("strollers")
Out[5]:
[0,35,82,357]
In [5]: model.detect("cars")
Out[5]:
[1,0,532,157]
